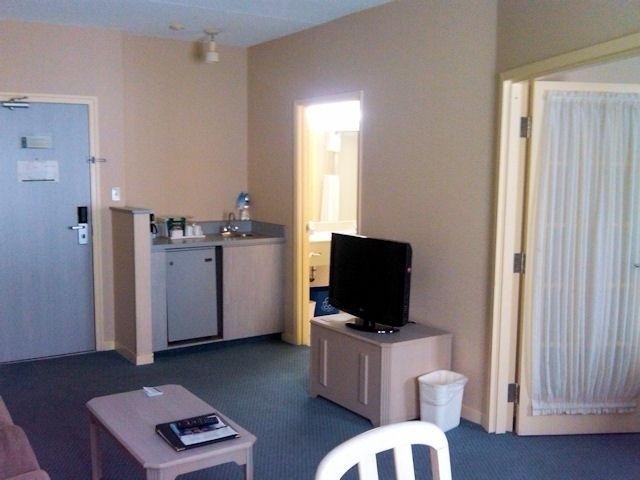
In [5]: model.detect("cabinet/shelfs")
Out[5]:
[164,246,220,343]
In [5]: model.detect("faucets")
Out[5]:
[228,212,237,229]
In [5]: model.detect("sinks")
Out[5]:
[220,232,254,239]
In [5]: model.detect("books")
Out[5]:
[154,411,241,452]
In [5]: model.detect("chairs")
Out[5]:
[312,419,453,480]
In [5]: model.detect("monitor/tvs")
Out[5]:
[328,233,412,333]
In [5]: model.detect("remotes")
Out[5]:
[178,417,219,428]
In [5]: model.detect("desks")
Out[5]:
[307,312,454,427]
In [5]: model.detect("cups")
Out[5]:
[185,224,203,236]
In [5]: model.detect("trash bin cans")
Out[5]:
[310,286,339,316]
[416,369,469,433]
